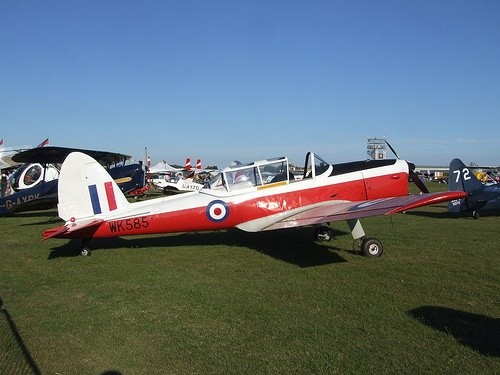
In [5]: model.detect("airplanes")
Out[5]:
[445,157,500,217]
[0,138,215,214]
[40,137,465,259]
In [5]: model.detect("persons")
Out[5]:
[0,166,17,197]
